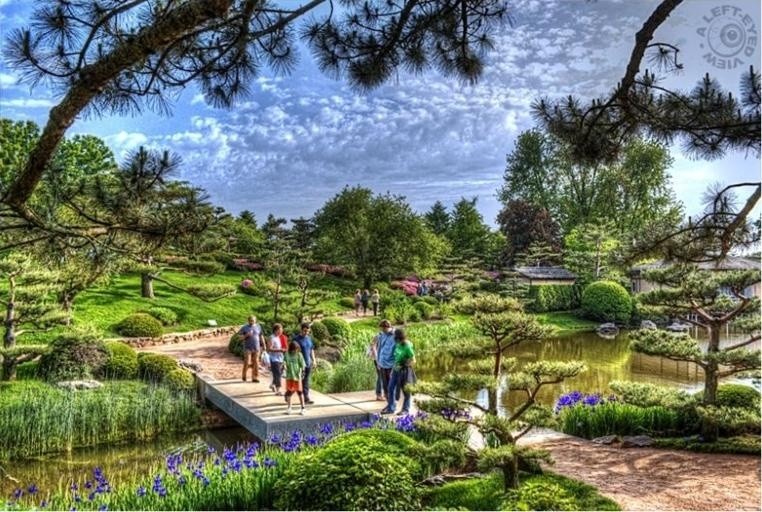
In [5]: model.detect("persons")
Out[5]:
[239,315,317,414]
[417,282,437,297]
[353,288,380,316]
[370,319,415,415]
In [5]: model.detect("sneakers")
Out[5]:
[304,400,314,405]
[242,377,260,382]
[286,407,306,415]
[269,384,288,402]
[376,395,410,415]
